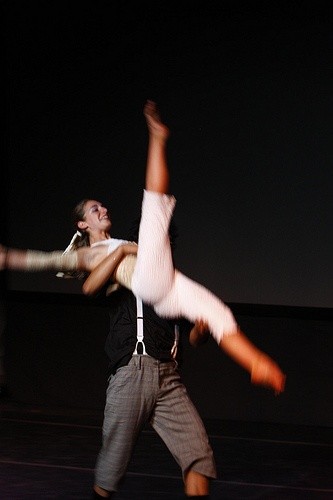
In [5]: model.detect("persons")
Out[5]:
[81,240,219,499]
[2,99,284,392]
